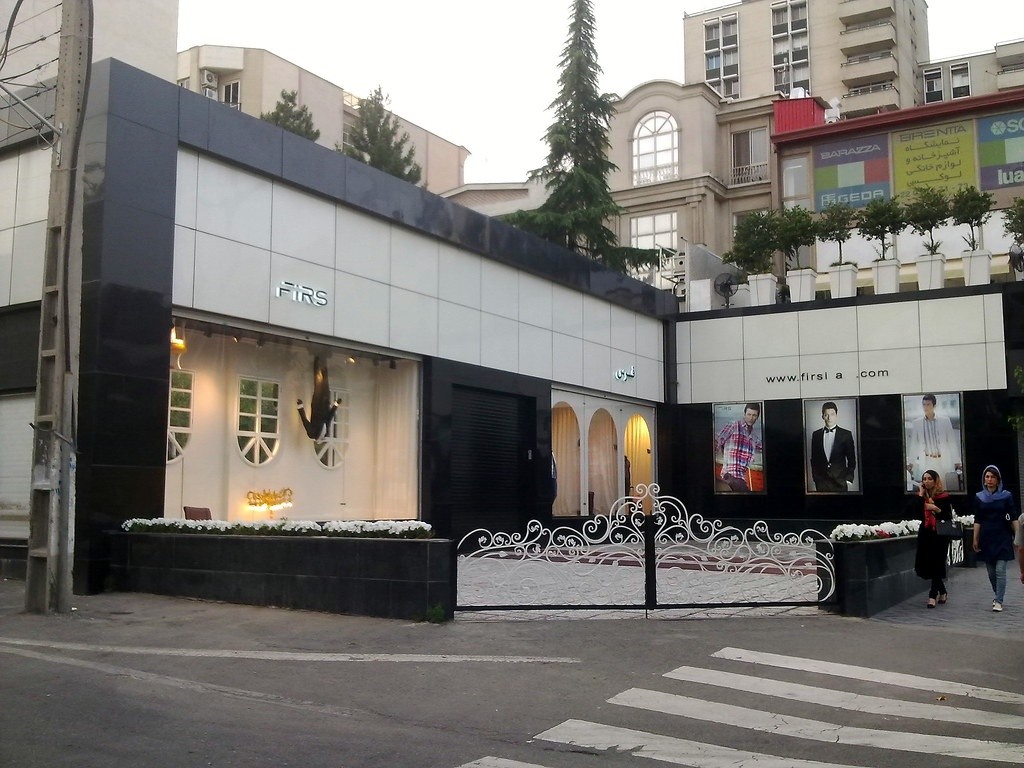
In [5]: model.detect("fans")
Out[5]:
[714,272,739,309]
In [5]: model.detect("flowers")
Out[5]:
[120,517,436,539]
[829,519,922,542]
[955,514,976,527]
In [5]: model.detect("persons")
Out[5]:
[908,394,962,490]
[972,464,1018,612]
[297,349,342,446]
[912,470,952,608]
[809,402,856,493]
[1014,513,1024,584]
[715,402,762,492]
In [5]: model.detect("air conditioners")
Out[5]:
[201,69,218,89]
[202,87,218,102]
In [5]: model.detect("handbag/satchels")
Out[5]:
[935,520,964,540]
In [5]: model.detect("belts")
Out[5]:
[926,455,941,458]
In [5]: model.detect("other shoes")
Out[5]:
[993,605,1004,611]
[992,601,996,606]
[927,598,936,609]
[938,592,948,603]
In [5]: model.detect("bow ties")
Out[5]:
[824,425,837,433]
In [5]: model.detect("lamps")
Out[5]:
[348,354,359,364]
[389,359,398,369]
[203,327,213,338]
[256,337,266,348]
[247,488,294,520]
[233,334,242,342]
[373,356,382,366]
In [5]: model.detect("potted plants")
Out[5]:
[813,201,860,299]
[721,209,783,306]
[772,205,819,303]
[904,186,954,291]
[855,195,908,295]
[949,184,999,286]
[999,195,1024,280]
